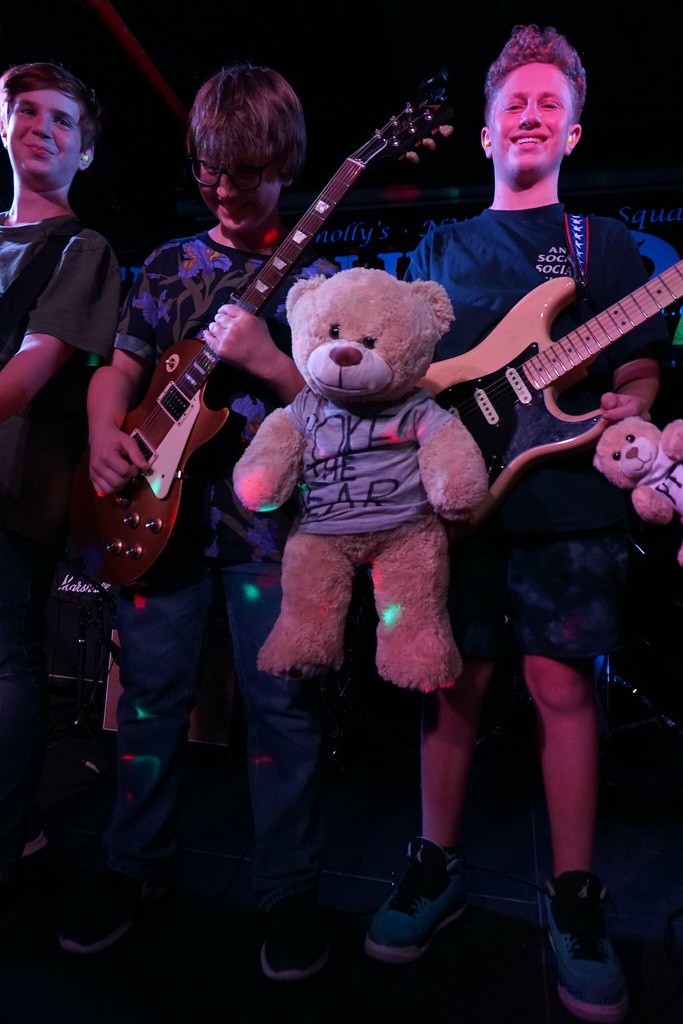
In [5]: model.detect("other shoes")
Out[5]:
[59,868,170,952]
[260,891,328,980]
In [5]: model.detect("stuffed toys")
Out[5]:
[233,267,489,695]
[594,416,683,565]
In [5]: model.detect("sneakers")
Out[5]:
[544,870,629,1023]
[365,836,468,963]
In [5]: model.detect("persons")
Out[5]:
[0,27,683,1024]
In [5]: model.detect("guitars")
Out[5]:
[413,257,683,542]
[57,72,461,600]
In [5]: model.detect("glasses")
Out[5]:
[188,148,270,192]
